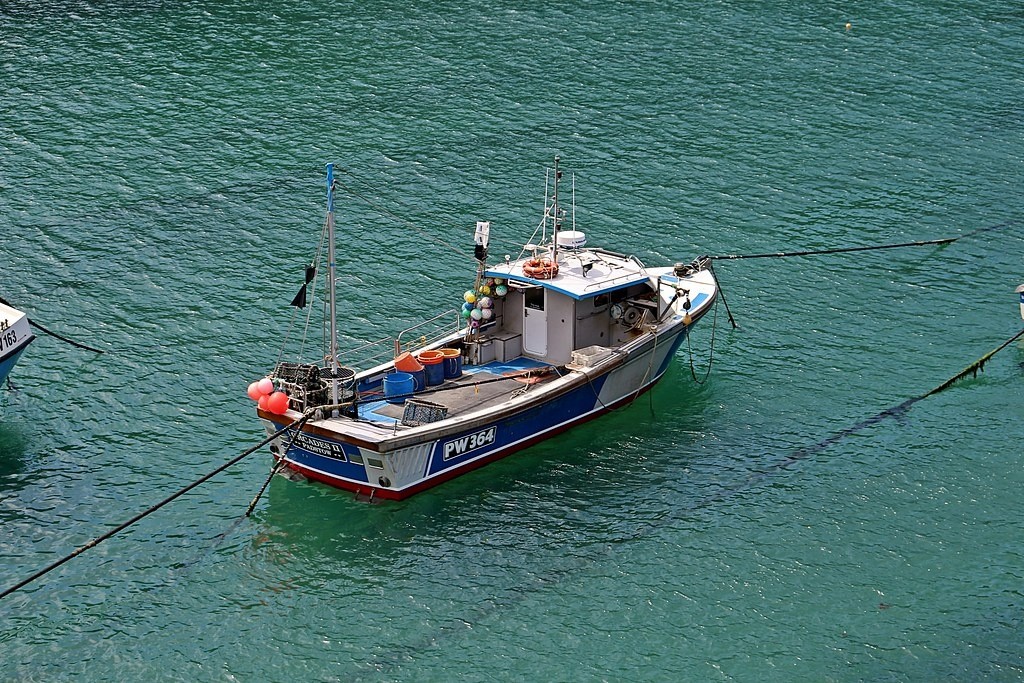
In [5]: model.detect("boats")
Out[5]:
[256,155,719,504]
[0,301,35,388]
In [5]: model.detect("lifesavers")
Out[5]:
[522,260,558,279]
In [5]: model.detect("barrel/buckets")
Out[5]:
[380,348,462,404]
